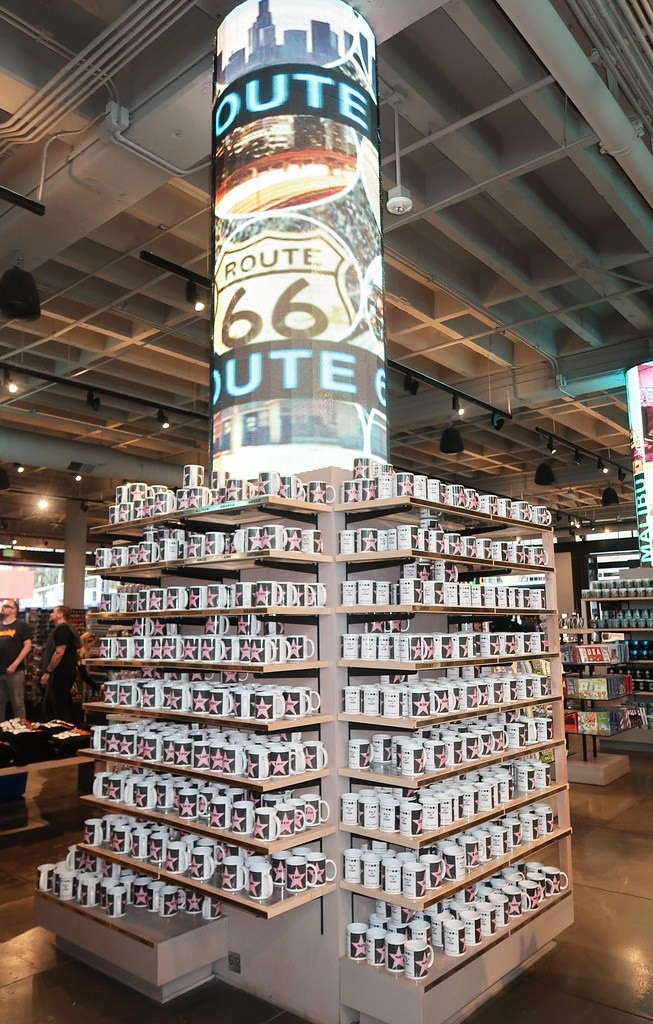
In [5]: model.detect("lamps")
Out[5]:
[71,471,82,482]
[3,369,18,394]
[0,265,41,323]
[535,463,556,487]
[602,488,620,507]
[184,281,207,312]
[452,394,465,416]
[157,407,170,429]
[13,462,26,474]
[403,374,420,396]
[557,511,596,536]
[492,411,507,432]
[547,436,626,482]
[86,391,100,411]
[439,427,465,456]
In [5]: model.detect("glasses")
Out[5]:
[3,604,14,609]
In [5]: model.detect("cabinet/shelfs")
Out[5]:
[30,493,335,1024]
[337,497,578,1024]
[581,597,653,754]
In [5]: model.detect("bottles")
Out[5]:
[610,669,653,692]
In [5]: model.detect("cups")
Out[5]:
[39,458,569,979]
[582,578,653,597]
[615,640,653,660]
[592,608,653,627]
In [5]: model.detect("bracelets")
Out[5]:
[45,669,52,674]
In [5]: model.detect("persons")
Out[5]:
[71,630,105,725]
[0,598,34,720]
[36,606,81,722]
